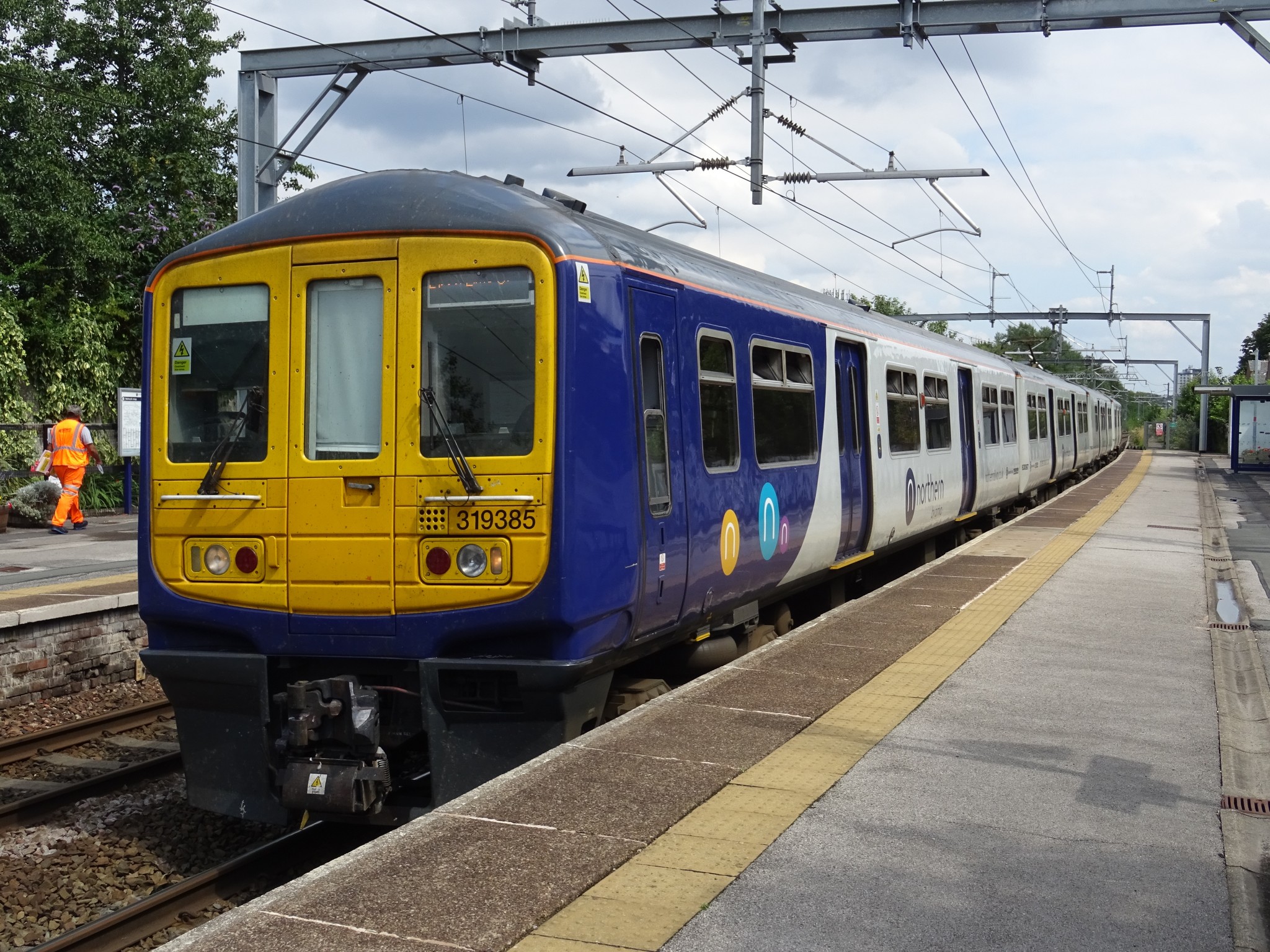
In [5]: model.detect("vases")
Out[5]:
[0,505,10,533]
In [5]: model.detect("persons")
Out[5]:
[43,404,103,534]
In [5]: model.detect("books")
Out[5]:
[35,457,58,479]
[38,450,53,463]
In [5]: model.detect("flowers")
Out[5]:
[0,488,12,509]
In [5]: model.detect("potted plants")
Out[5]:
[7,479,63,528]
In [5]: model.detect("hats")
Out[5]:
[67,405,85,416]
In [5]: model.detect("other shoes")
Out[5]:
[51,524,68,534]
[73,520,88,530]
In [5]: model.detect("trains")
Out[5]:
[137,168,1123,822]
